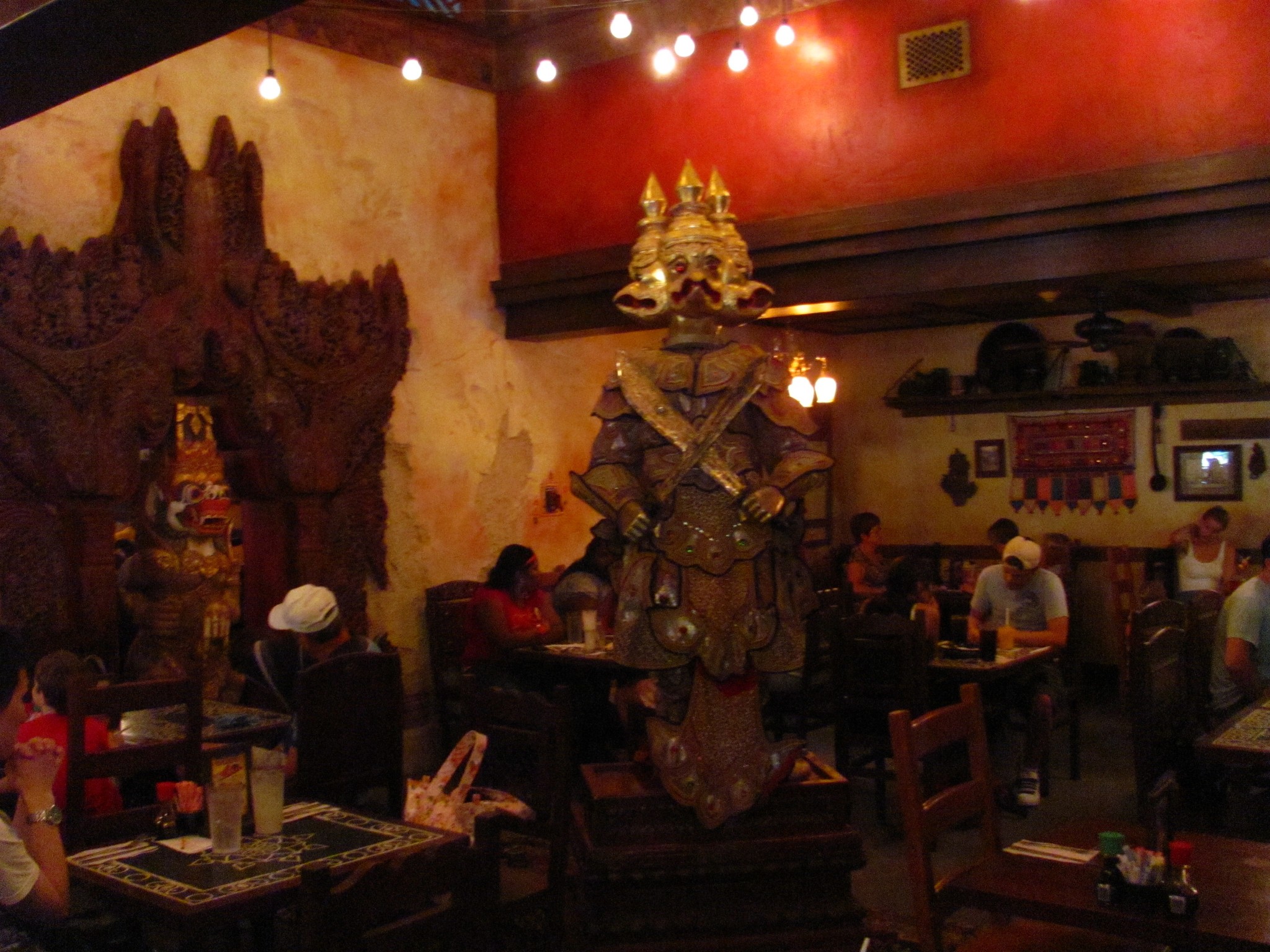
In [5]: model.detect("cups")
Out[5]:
[208,785,242,854]
[980,629,997,661]
[249,770,285,835]
[567,614,582,643]
[997,626,1015,650]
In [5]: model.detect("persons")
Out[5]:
[572,157,832,823]
[844,512,919,605]
[987,518,1018,556]
[553,534,621,641]
[1167,505,1238,644]
[265,583,406,821]
[967,536,1070,808]
[1209,532,1270,721]
[0,628,143,952]
[116,399,250,706]
[471,542,577,703]
[229,566,297,715]
[10,650,123,818]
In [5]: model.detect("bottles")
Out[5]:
[1096,858,1125,909]
[1159,866,1201,920]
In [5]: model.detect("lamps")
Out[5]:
[763,318,838,413]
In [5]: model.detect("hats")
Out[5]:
[1002,536,1042,571]
[267,583,341,635]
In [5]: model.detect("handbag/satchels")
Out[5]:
[405,729,536,905]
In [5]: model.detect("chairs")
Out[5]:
[832,605,965,829]
[886,683,1173,952]
[274,804,504,952]
[1186,588,1224,702]
[65,659,207,857]
[460,673,584,952]
[1003,571,1086,782]
[1123,596,1194,835]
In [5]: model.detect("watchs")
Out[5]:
[26,804,63,825]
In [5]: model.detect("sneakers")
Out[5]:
[1017,771,1041,805]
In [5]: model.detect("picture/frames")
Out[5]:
[1172,442,1244,502]
[973,437,1007,478]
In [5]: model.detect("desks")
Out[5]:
[115,697,297,772]
[1194,690,1270,774]
[923,642,1053,677]
[948,815,1270,952]
[64,797,477,952]
